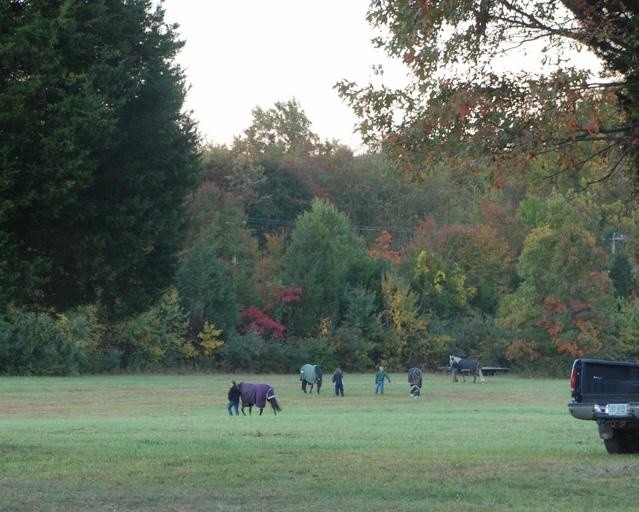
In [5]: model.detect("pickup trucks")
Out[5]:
[568,359,638,455]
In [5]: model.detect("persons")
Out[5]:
[451,358,458,381]
[332,368,344,397]
[227,380,241,416]
[375,365,391,394]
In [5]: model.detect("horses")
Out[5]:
[408,366,423,397]
[300,363,323,395]
[449,355,486,384]
[237,381,281,416]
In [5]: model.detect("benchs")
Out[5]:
[436,367,510,375]
